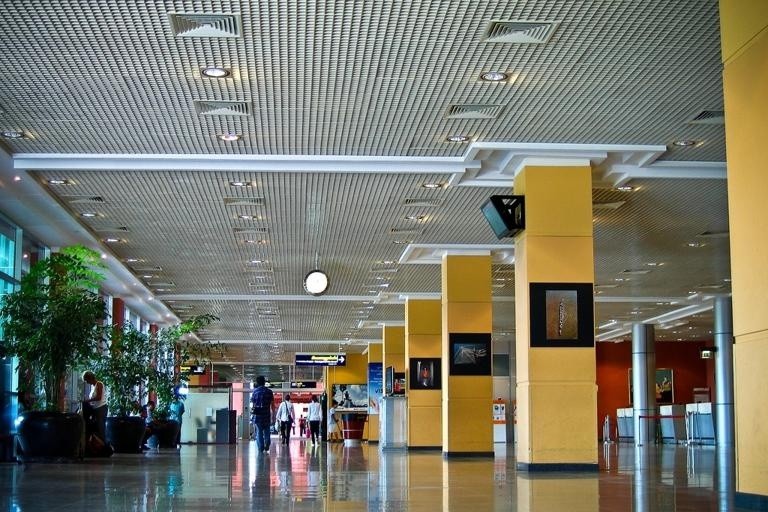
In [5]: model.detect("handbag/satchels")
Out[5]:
[288,415,293,422]
[251,407,257,414]
[274,421,280,432]
[306,428,310,437]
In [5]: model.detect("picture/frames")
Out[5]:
[627,367,675,405]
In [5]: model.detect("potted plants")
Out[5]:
[93,319,156,454]
[147,312,228,449]
[0,241,113,460]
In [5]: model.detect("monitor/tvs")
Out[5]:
[479,196,516,240]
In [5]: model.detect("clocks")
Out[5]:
[302,252,330,296]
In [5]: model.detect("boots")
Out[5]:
[105,442,115,458]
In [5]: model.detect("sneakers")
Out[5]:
[259,449,270,456]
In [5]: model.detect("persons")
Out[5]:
[130,391,185,450]
[78,370,108,453]
[250,375,295,453]
[298,394,323,444]
[328,399,341,443]
